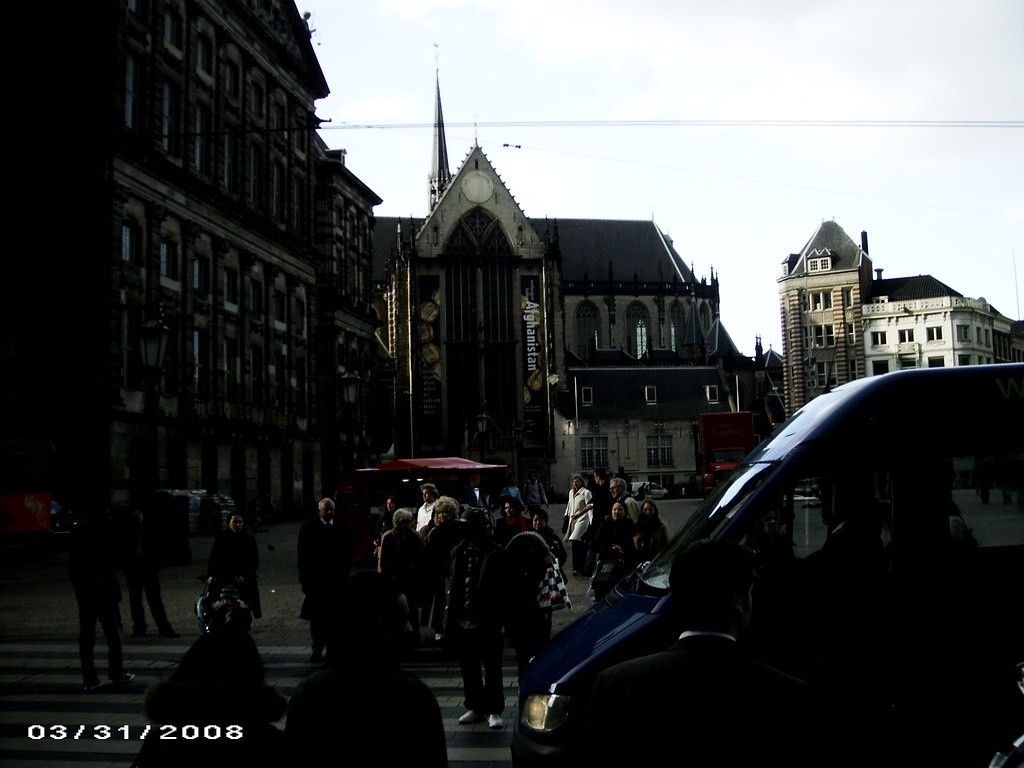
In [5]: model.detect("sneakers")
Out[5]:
[459,710,486,723]
[486,713,504,728]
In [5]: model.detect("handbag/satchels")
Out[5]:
[536,547,572,611]
[591,559,618,590]
[445,544,473,613]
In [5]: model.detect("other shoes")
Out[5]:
[109,672,131,681]
[420,626,432,640]
[434,631,446,641]
[83,675,103,692]
[132,625,146,637]
[159,629,180,638]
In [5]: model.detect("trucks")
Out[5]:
[696,411,757,491]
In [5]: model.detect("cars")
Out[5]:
[624,481,669,500]
[142,489,243,533]
[0,482,82,567]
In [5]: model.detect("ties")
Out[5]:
[325,523,332,529]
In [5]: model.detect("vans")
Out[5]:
[513,360,1024,768]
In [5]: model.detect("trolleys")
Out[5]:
[194,575,245,640]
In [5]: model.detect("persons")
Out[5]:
[129,626,285,768]
[573,467,668,603]
[206,511,262,631]
[296,498,358,664]
[68,506,135,689]
[119,501,181,639]
[815,453,1024,554]
[582,537,809,768]
[563,473,594,577]
[743,490,796,560]
[372,472,568,728]
[283,599,449,768]
